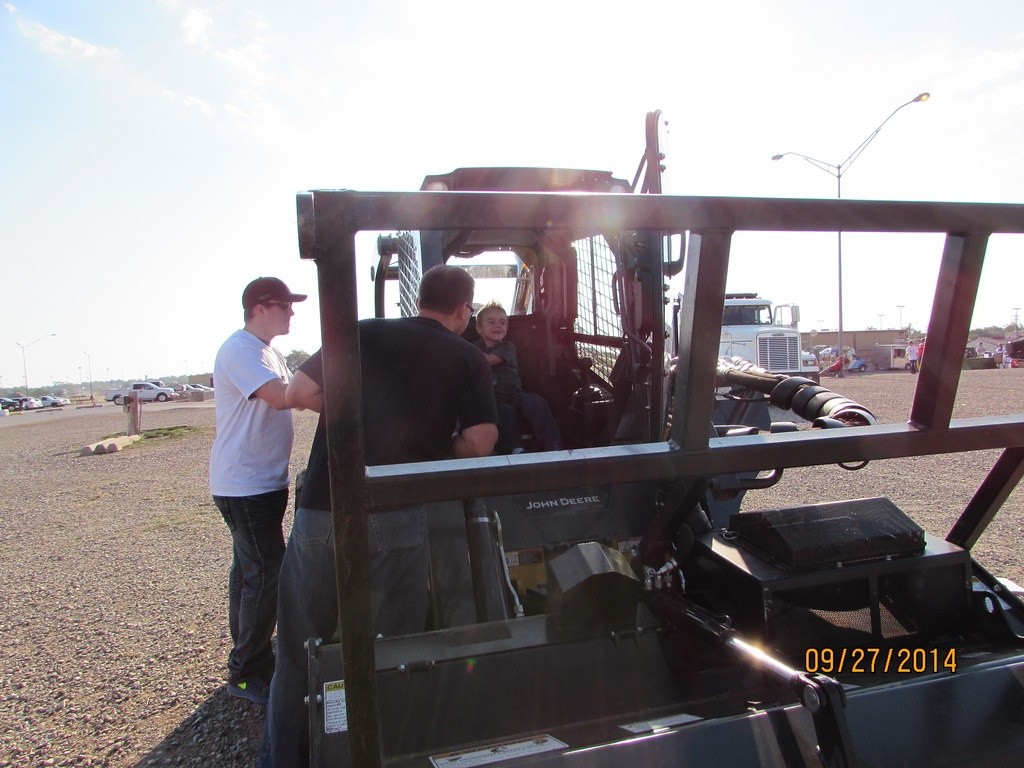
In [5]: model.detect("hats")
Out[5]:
[242,277,308,309]
[837,356,842,360]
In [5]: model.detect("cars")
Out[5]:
[0,396,70,412]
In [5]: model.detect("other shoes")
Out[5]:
[227,673,271,704]
[262,656,276,684]
[509,451,535,456]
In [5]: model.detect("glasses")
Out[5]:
[268,301,292,312]
[459,300,475,318]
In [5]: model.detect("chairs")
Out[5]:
[465,308,594,443]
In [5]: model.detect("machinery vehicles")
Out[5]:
[296,167,1024,768]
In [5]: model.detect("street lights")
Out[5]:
[16,333,57,397]
[772,93,929,376]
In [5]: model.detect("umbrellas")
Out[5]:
[818,348,839,362]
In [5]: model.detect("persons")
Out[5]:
[819,356,842,375]
[844,356,849,373]
[208,276,307,705]
[996,343,1006,368]
[19,398,24,413]
[918,338,925,365]
[906,340,918,374]
[256,266,524,768]
[977,342,985,354]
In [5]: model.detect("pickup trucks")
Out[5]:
[104,381,214,405]
[672,292,820,384]
